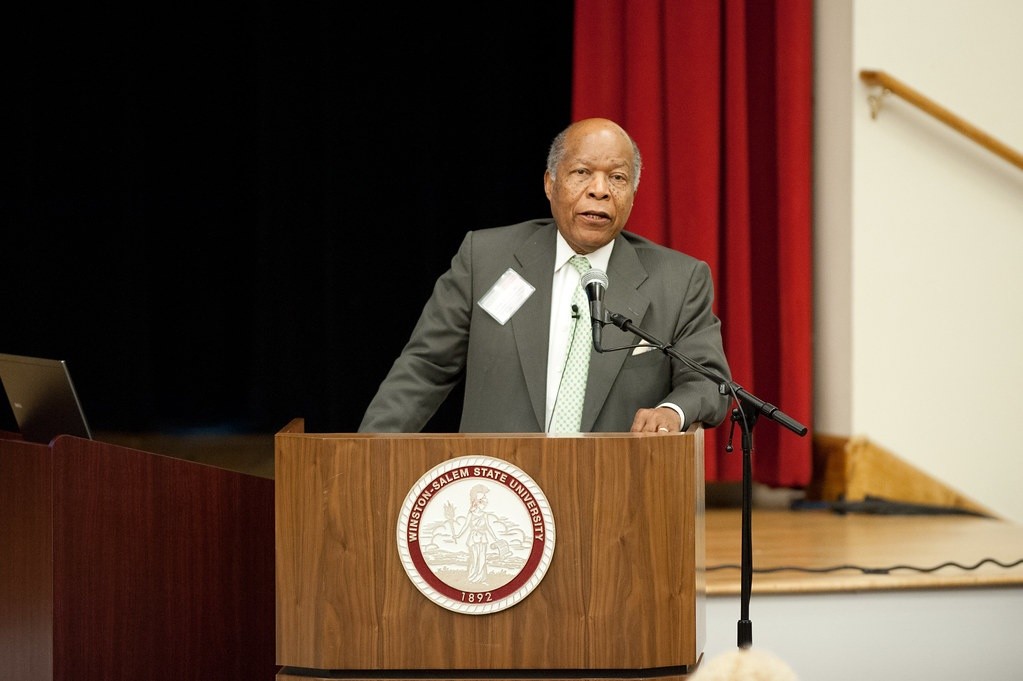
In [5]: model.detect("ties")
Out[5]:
[548,252,593,436]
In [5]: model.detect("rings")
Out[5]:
[659,428,668,432]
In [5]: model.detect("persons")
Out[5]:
[358,118,733,434]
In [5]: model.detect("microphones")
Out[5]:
[572,305,580,318]
[581,267,609,349]
